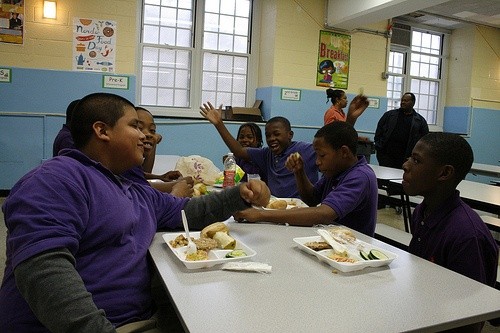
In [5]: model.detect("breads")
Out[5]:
[270,200,287,210]
[200,222,229,239]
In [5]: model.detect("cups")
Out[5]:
[247,174,260,182]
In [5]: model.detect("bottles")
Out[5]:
[223,153,236,189]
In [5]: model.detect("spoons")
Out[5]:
[318,229,348,255]
[181,210,197,254]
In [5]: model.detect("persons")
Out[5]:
[0,92,270,333]
[198,93,370,199]
[122,107,195,198]
[141,135,184,182]
[323,89,349,125]
[52,99,81,157]
[374,92,430,170]
[236,122,264,182]
[10,12,21,29]
[402,132,499,333]
[232,121,379,238]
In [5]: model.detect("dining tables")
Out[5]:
[147,163,500,333]
